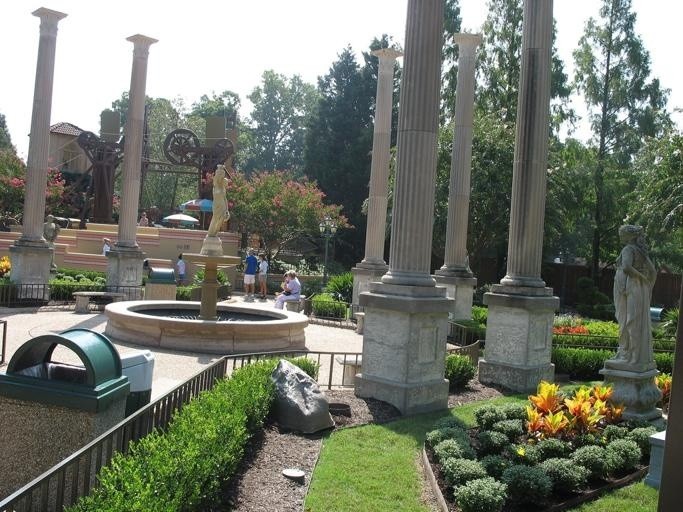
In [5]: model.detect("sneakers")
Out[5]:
[243,294,267,300]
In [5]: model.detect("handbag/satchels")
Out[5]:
[283,291,290,296]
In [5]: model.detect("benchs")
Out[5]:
[72,291,126,313]
[286,294,306,313]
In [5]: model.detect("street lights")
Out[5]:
[319,212,339,287]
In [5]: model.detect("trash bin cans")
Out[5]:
[144,267,177,300]
[0,327,155,512]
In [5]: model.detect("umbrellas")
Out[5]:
[162,197,216,230]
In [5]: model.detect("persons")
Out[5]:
[175,253,186,288]
[139,212,148,227]
[606,222,659,374]
[102,237,112,257]
[43,214,60,268]
[275,270,301,310]
[242,248,269,300]
